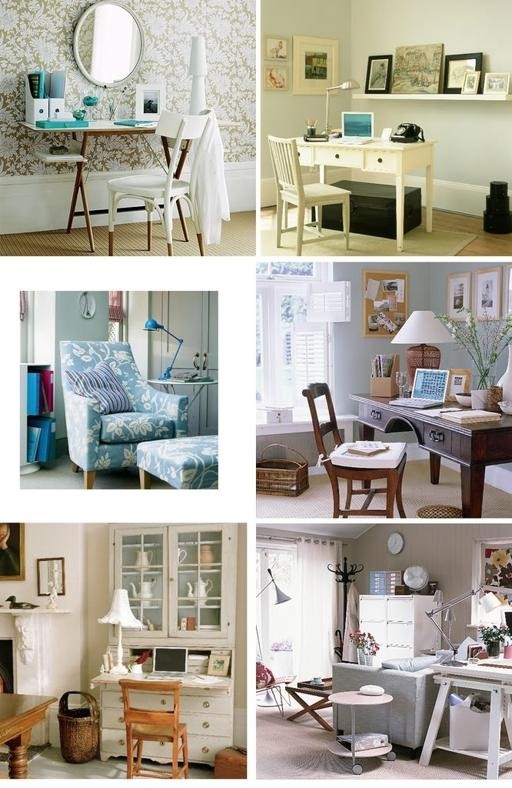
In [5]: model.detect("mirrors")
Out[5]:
[74,1,146,88]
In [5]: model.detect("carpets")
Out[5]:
[262,205,479,256]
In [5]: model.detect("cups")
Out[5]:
[273,640,292,649]
[306,126,316,135]
[504,644,512,659]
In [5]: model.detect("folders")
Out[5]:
[28,417,56,461]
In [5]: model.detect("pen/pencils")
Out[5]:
[306,120,317,128]
[194,675,204,680]
[440,409,470,413]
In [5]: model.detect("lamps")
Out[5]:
[140,318,184,380]
[256,567,291,708]
[424,584,502,653]
[391,311,455,390]
[322,79,361,137]
[99,589,146,675]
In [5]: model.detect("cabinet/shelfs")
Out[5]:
[108,523,237,648]
[359,594,442,669]
[89,646,235,770]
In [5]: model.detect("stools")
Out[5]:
[137,434,218,488]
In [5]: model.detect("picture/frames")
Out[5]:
[447,265,511,322]
[264,34,339,96]
[362,270,408,338]
[443,52,510,96]
[365,55,392,93]
[135,82,167,120]
[0,522,25,580]
[445,369,472,403]
[207,656,231,677]
[38,557,65,596]
[467,532,512,627]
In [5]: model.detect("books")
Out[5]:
[348,440,389,457]
[27,427,41,462]
[442,409,501,425]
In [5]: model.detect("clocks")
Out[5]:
[386,532,405,555]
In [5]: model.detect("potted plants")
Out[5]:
[436,307,511,411]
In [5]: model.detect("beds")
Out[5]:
[147,378,218,410]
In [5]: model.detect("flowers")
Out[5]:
[478,623,510,642]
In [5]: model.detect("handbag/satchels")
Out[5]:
[449,693,489,751]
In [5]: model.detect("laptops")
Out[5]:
[330,111,374,145]
[146,647,188,680]
[390,368,451,409]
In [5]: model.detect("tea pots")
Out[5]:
[178,547,187,564]
[187,576,214,606]
[127,580,154,607]
[135,550,153,572]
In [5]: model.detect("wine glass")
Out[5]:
[395,370,409,398]
[79,85,99,121]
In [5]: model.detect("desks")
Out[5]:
[0,691,58,778]
[420,664,512,780]
[349,392,512,518]
[20,119,192,252]
[286,134,439,255]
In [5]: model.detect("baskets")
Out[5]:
[57,691,101,764]
[256,443,310,496]
[418,504,465,519]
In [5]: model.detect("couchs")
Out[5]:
[331,655,451,758]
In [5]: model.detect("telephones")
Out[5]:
[391,122,420,143]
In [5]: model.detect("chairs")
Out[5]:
[256,675,297,719]
[107,109,213,255]
[268,135,353,256]
[302,381,408,518]
[120,679,190,780]
[60,339,189,488]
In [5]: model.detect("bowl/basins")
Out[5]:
[313,675,322,682]
[455,391,472,407]
[497,400,512,415]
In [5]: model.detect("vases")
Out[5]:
[489,640,500,656]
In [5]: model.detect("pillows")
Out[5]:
[62,361,136,413]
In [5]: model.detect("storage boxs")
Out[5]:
[214,745,247,779]
